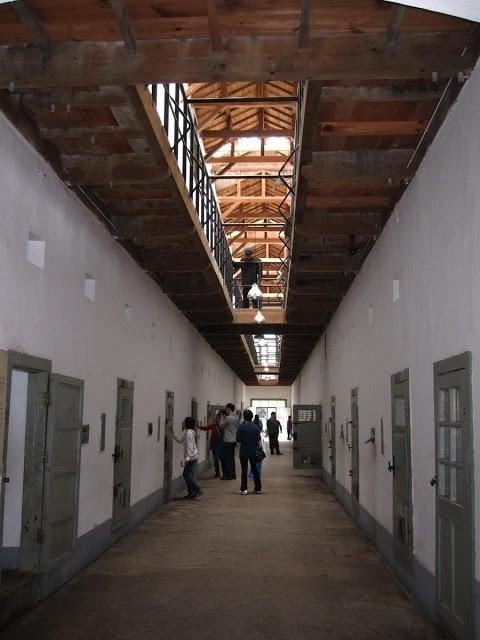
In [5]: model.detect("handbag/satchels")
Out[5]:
[256,450,265,463]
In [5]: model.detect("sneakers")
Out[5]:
[240,490,247,494]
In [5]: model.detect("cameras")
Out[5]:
[220,409,226,414]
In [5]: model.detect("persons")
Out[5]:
[170,417,203,499]
[232,248,263,308]
[199,402,283,495]
[286,416,293,440]
